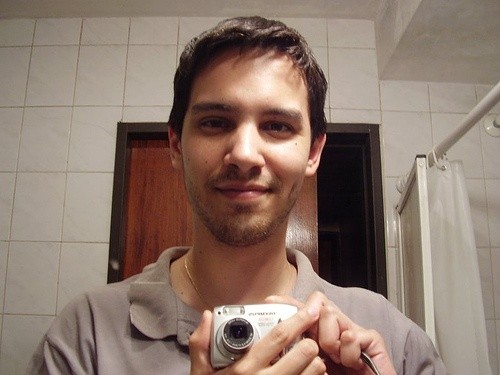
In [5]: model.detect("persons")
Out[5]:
[27,15,448,375]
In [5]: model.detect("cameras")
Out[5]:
[210,304,302,368]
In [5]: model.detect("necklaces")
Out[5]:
[184,254,294,312]
